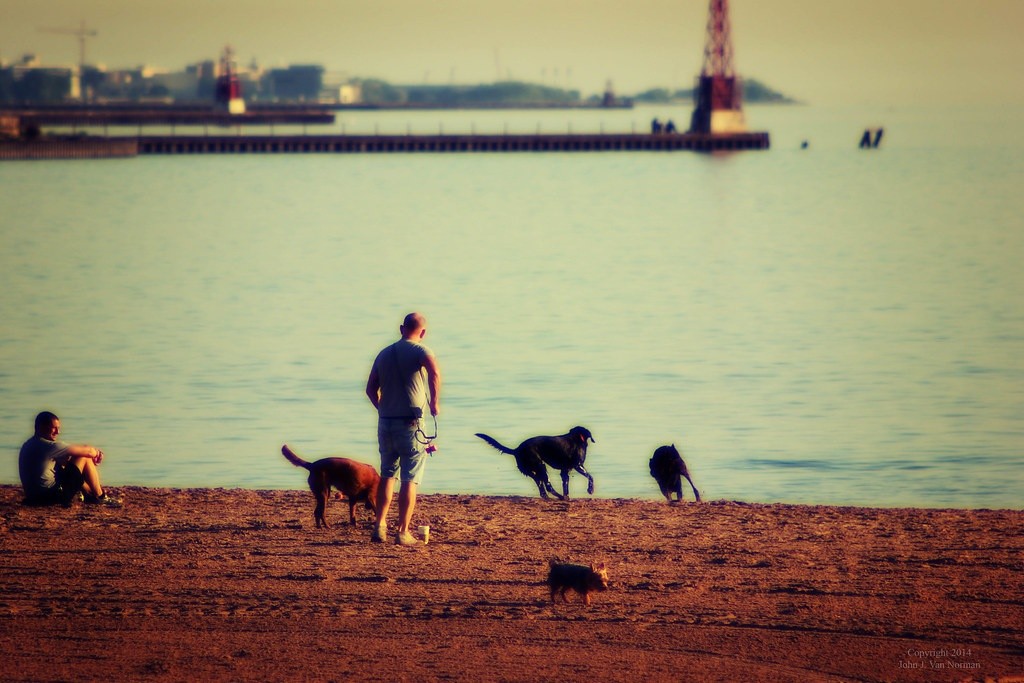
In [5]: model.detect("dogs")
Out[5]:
[473,425,596,498]
[280,443,380,529]
[544,553,609,605]
[648,443,701,501]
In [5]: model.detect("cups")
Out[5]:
[418,526,430,543]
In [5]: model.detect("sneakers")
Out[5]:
[84,493,123,507]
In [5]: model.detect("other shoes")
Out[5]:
[395,530,424,546]
[371,530,387,543]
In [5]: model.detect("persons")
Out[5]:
[365,312,442,544]
[18,411,123,508]
[652,118,678,135]
